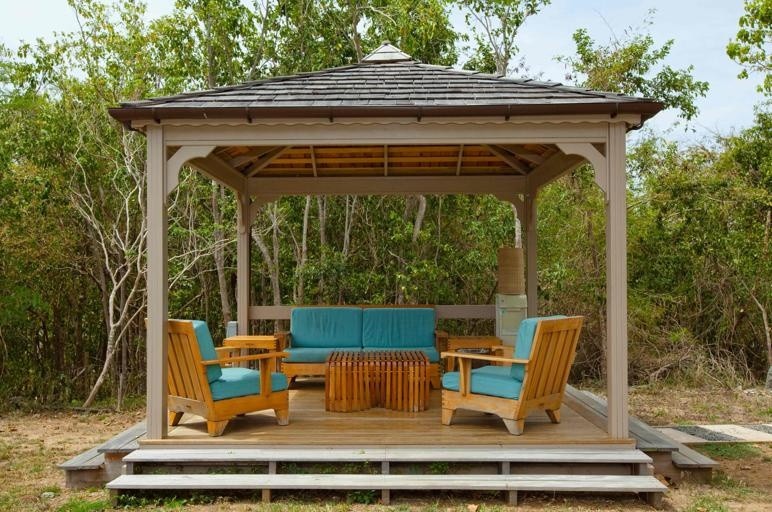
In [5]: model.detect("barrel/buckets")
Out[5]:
[499,247,525,295]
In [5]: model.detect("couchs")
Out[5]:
[441,316,584,436]
[144,317,291,437]
[274,304,448,389]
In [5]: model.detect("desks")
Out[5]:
[447,336,502,371]
[223,336,276,372]
